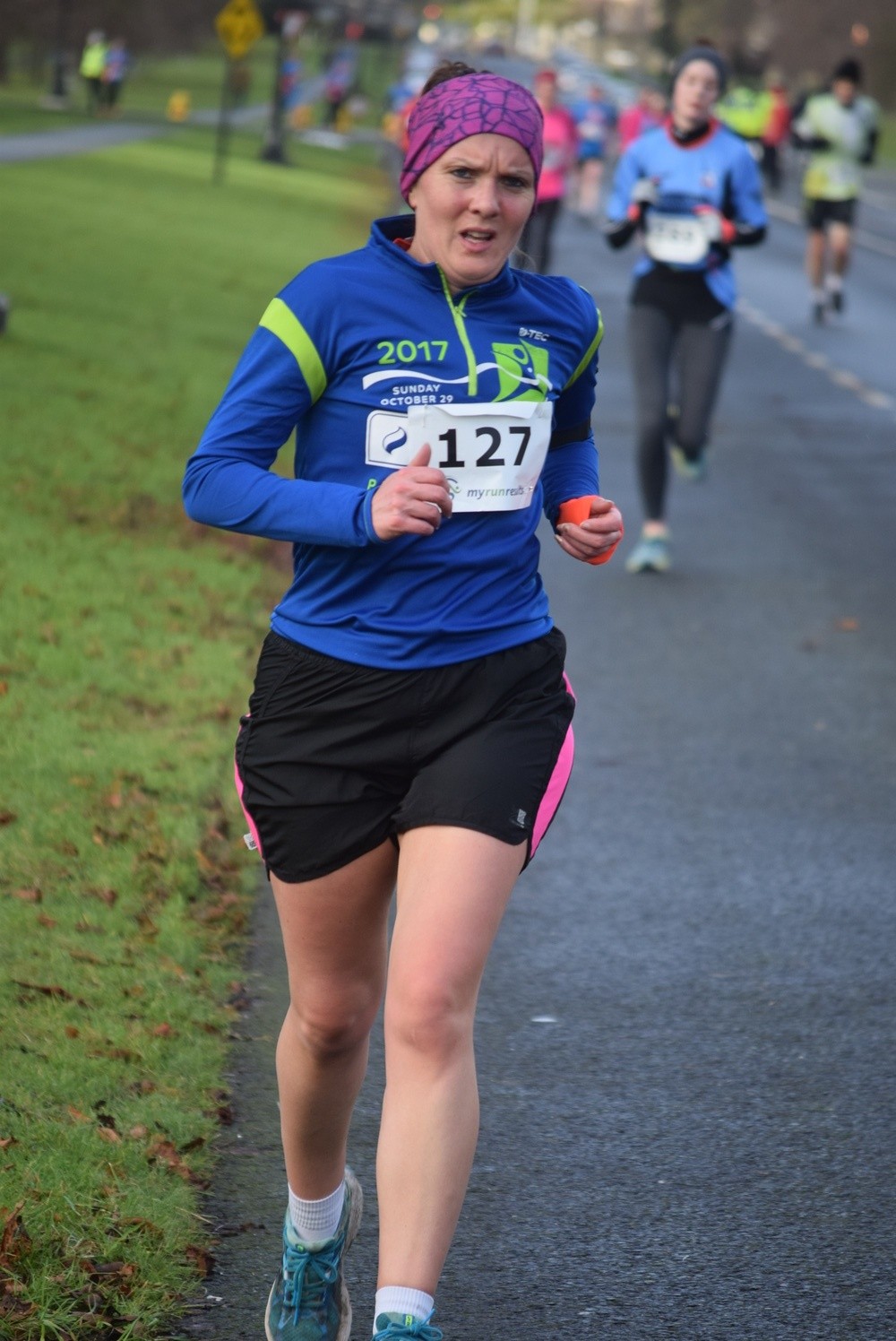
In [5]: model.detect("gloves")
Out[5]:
[692,205,736,245]
[628,177,661,222]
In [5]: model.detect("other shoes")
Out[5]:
[625,537,672,574]
[671,445,707,484]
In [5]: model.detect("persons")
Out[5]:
[183,59,624,1341]
[78,1,884,323]
[603,46,770,571]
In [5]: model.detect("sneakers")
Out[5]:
[370,1307,443,1341]
[265,1166,364,1341]
